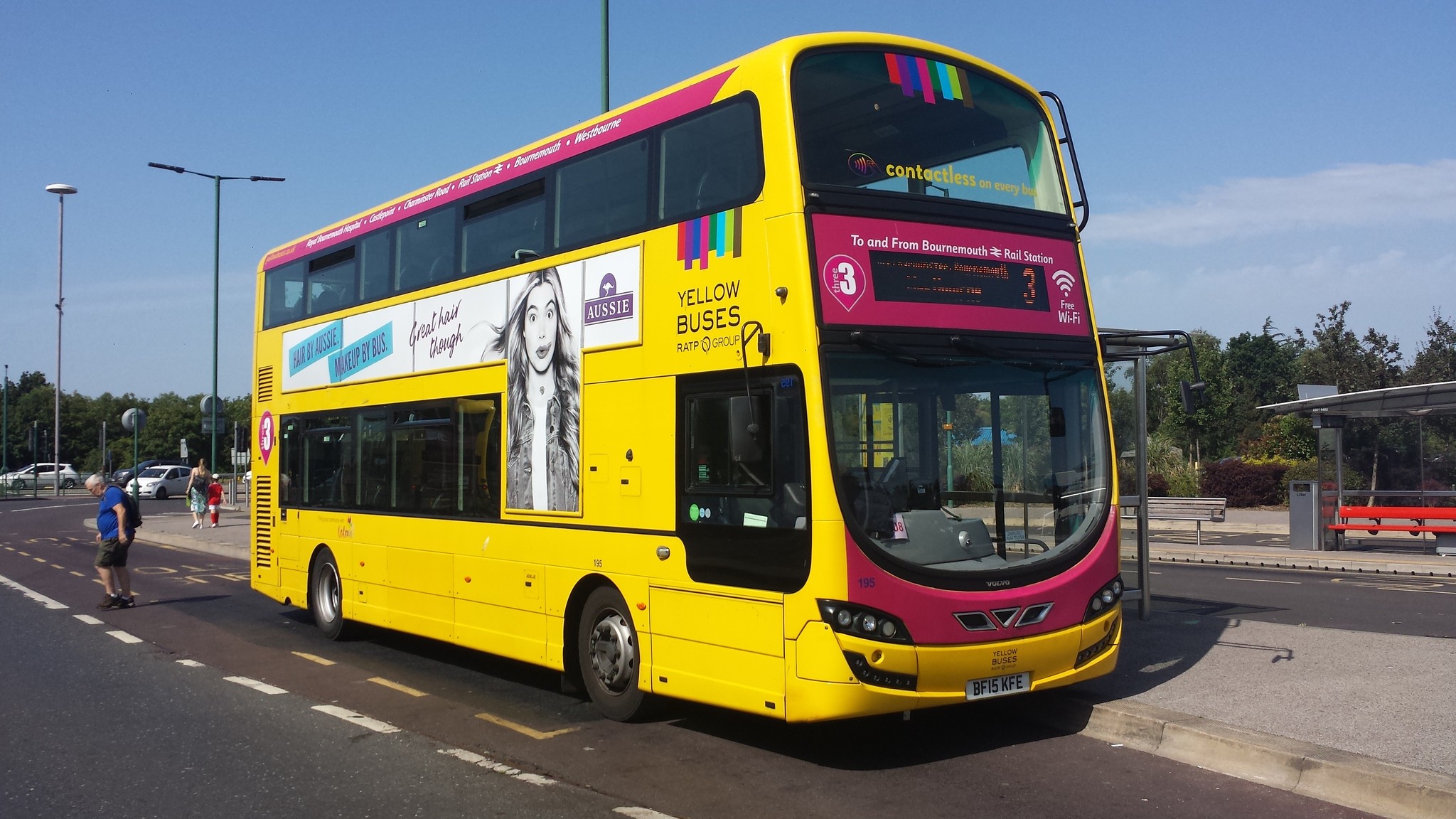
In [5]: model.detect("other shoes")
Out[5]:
[216,523,219,526]
[199,525,203,529]
[212,523,217,528]
[192,521,199,528]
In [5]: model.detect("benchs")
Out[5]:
[1119,497,1227,542]
[1329,506,1456,551]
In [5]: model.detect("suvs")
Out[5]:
[111,459,192,488]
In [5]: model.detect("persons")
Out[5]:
[185,458,211,529]
[482,265,586,514]
[84,472,144,611]
[208,473,227,528]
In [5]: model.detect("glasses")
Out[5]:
[88,481,100,492]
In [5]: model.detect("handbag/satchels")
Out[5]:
[191,476,206,490]
[186,494,191,507]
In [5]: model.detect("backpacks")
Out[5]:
[102,483,143,528]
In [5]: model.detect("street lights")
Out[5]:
[147,161,286,471]
[45,184,78,496]
[244,30,1213,728]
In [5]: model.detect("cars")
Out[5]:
[126,465,192,501]
[0,462,81,490]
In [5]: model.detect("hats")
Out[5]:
[212,474,220,479]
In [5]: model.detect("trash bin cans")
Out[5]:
[1289,480,1335,550]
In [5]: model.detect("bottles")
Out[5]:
[185,497,190,506]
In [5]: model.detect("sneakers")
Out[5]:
[97,594,122,609]
[112,595,135,608]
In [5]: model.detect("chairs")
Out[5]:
[290,253,452,317]
[331,463,476,518]
[694,168,733,212]
